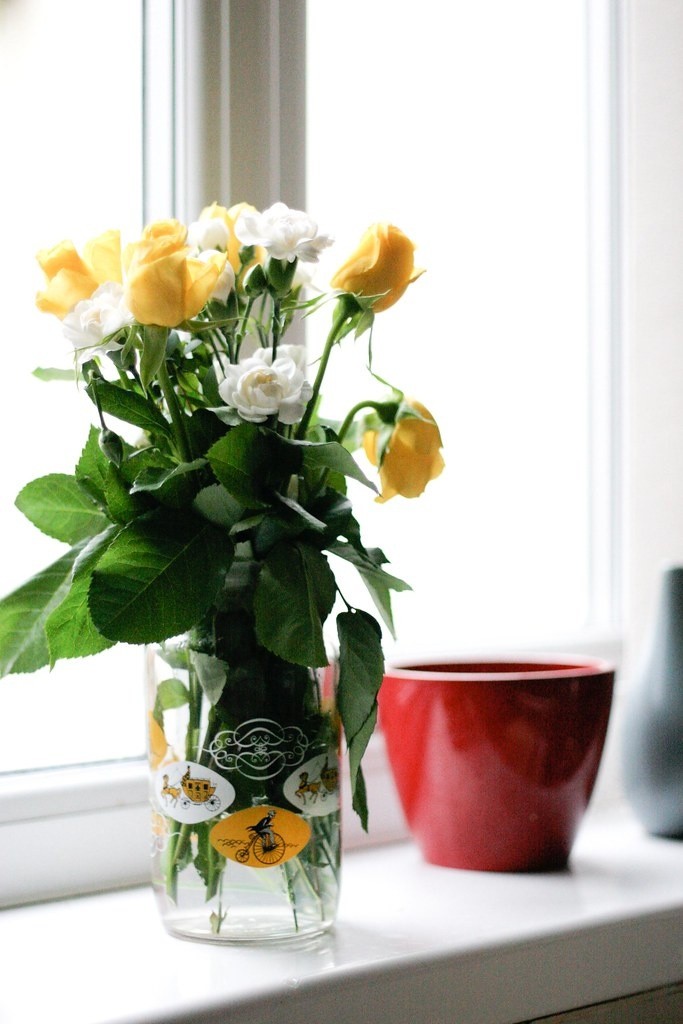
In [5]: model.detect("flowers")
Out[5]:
[0,201,449,939]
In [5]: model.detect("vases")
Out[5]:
[379,658,618,869]
[141,630,342,941]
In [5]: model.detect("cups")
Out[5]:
[379,656,615,871]
